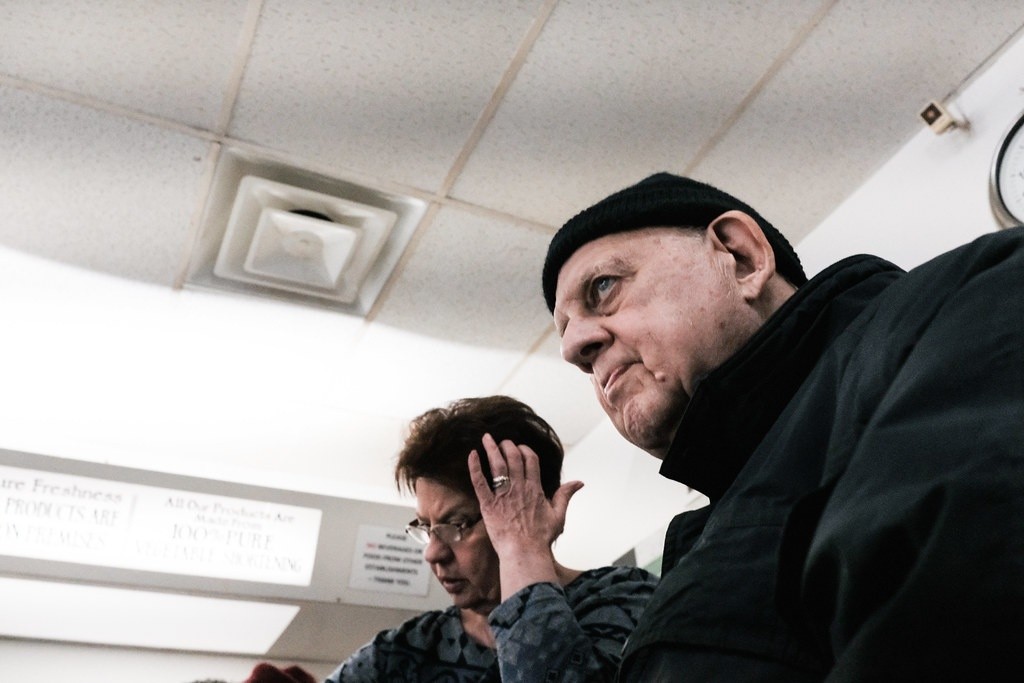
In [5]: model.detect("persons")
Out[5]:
[326,397,664,682]
[539,170,1024,682]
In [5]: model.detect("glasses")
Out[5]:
[405,515,483,545]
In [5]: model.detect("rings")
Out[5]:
[490,474,506,488]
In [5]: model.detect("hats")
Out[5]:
[542,170,807,318]
